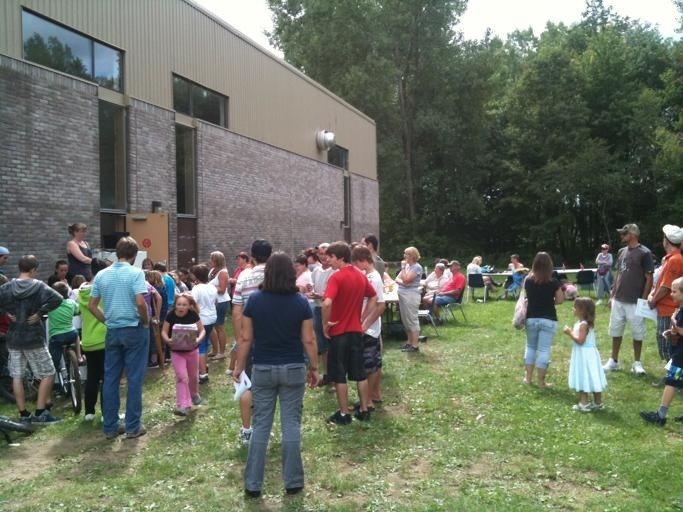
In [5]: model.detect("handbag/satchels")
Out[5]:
[513,276,528,330]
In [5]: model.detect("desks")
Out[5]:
[382,278,426,325]
[481,272,514,276]
[552,268,598,298]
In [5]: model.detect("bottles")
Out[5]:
[385,286,389,295]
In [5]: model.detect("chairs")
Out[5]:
[573,269,594,297]
[468,274,490,302]
[440,285,470,326]
[418,290,440,337]
[553,271,568,281]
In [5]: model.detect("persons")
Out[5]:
[232,252,321,497]
[423,258,466,324]
[501,254,524,299]
[594,222,683,428]
[521,252,564,388]
[161,240,272,441]
[294,233,392,422]
[395,247,423,353]
[467,256,502,292]
[564,297,608,412]
[0,223,168,438]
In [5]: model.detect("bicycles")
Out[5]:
[0,341,43,402]
[58,344,81,413]
[0,416,35,442]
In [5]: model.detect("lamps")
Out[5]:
[316,129,335,151]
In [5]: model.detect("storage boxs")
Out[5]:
[171,323,198,351]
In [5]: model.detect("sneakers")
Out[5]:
[603,358,618,371]
[199,352,225,384]
[595,299,602,305]
[318,379,336,392]
[631,361,645,375]
[324,400,384,425]
[398,343,420,353]
[18,408,147,439]
[147,360,168,369]
[174,393,202,415]
[238,425,252,445]
[640,410,666,428]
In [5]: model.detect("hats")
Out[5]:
[447,260,460,267]
[601,243,610,250]
[615,223,640,237]
[662,224,683,244]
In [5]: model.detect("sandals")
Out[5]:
[572,402,605,412]
